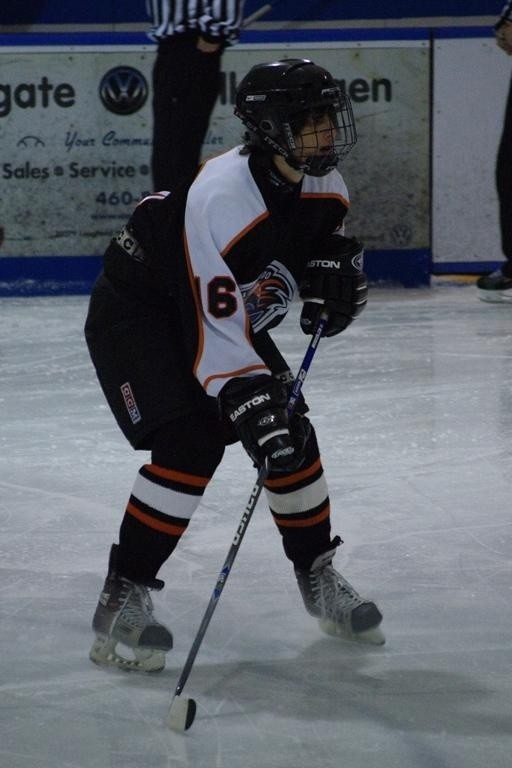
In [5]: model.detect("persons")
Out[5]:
[473,1,511,291]
[77,52,388,654]
[142,1,276,194]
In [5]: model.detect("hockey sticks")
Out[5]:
[165,300,330,727]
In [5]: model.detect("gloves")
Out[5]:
[296,238,371,340]
[216,371,305,474]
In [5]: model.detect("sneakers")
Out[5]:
[475,263,512,290]
[279,530,384,633]
[91,543,175,652]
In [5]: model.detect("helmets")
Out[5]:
[233,55,361,179]
